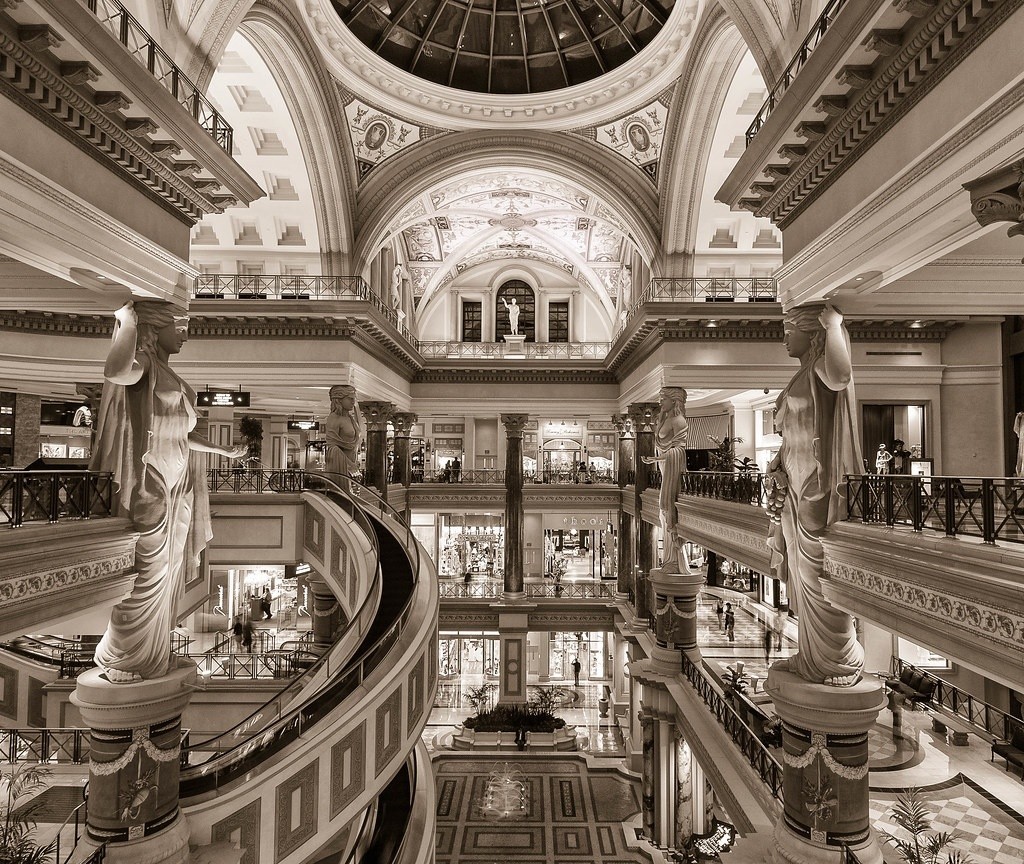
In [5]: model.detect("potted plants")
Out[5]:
[237,414,264,474]
[705,432,760,502]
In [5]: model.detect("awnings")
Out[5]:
[685,415,728,449]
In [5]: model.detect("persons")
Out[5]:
[86,299,248,688]
[715,598,734,642]
[233,617,257,653]
[292,597,296,601]
[722,561,729,571]
[502,297,520,335]
[287,460,299,468]
[244,586,255,599]
[324,385,361,490]
[391,265,403,309]
[889,438,912,475]
[574,632,583,647]
[571,658,581,687]
[764,609,787,664]
[876,444,893,479]
[641,388,691,574]
[263,588,273,619]
[768,301,865,687]
[621,269,631,309]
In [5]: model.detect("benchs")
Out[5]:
[928,712,973,747]
[990,725,1024,783]
[884,666,938,711]
